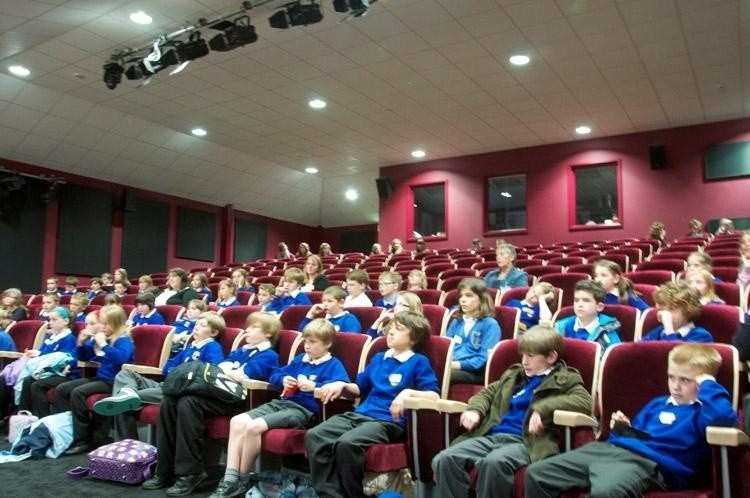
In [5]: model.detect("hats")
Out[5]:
[134,292,156,309]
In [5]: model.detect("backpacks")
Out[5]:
[68,439,158,485]
[246,469,319,497]
[163,360,248,403]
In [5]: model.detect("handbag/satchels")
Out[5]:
[12,411,74,458]
[0,354,30,386]
[7,410,39,444]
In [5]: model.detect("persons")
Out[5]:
[431,218,750,498]
[1,276,135,456]
[92,239,438,497]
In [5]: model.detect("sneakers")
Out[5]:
[210,479,246,498]
[64,442,89,455]
[93,389,141,416]
[143,475,177,489]
[166,471,207,497]
[240,471,258,489]
